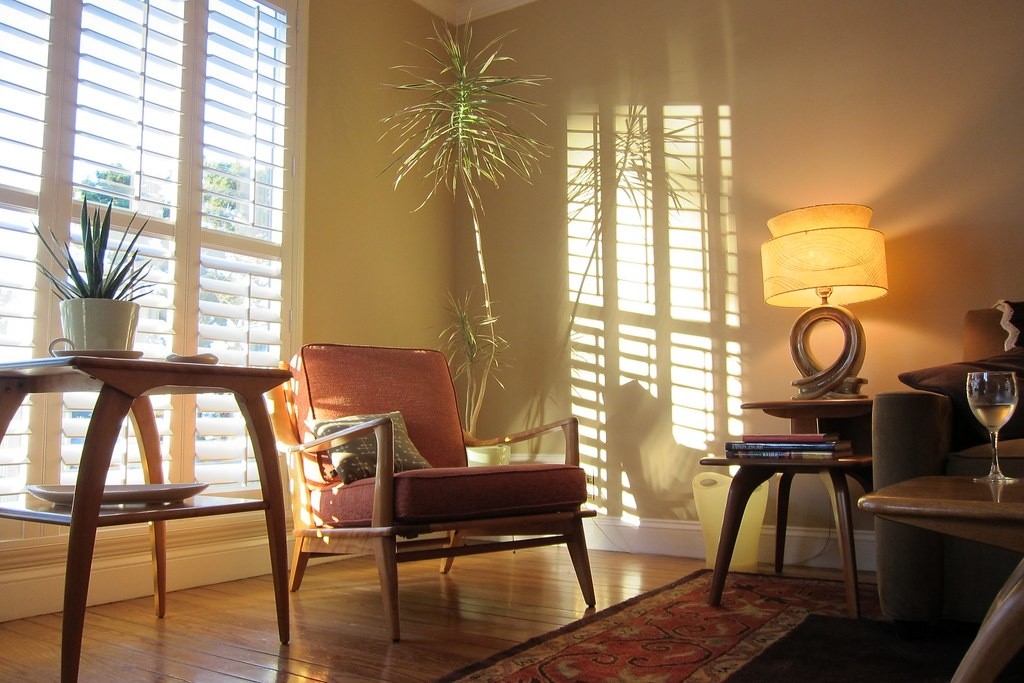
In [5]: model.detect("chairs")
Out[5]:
[266,342,600,642]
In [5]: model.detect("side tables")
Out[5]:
[698,397,874,619]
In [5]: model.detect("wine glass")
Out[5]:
[966,371,1019,485]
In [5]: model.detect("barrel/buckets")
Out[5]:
[692,471,770,574]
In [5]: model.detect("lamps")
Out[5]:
[760,202,888,400]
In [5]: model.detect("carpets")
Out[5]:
[428,569,1024,683]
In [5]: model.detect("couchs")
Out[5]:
[871,299,1024,620]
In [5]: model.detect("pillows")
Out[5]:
[305,410,435,484]
[898,347,1024,453]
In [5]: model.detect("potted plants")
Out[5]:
[375,5,557,466]
[31,194,157,359]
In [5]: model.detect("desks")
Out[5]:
[0,356,293,683]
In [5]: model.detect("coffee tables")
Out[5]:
[857,475,1024,683]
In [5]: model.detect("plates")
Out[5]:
[26,483,209,505]
[52,350,143,359]
